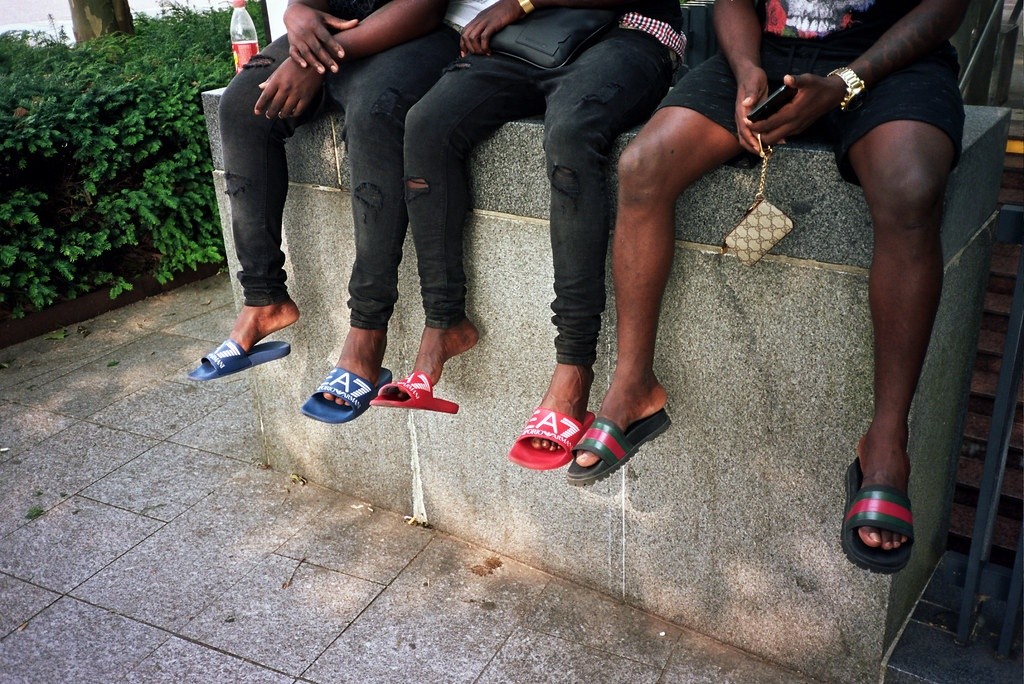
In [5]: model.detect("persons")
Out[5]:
[369,0,686,470]
[185,0,459,422]
[567,0,972,576]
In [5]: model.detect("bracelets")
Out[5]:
[518,0,535,14]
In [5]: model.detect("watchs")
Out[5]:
[827,67,867,113]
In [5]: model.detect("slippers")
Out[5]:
[299,367,393,424]
[369,370,459,415]
[508,410,596,472]
[839,456,914,575]
[566,408,671,487]
[187,339,291,381]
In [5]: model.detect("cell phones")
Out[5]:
[747,85,796,123]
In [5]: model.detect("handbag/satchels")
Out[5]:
[485,4,620,71]
[719,134,795,267]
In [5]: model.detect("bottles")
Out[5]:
[230,0,260,75]
